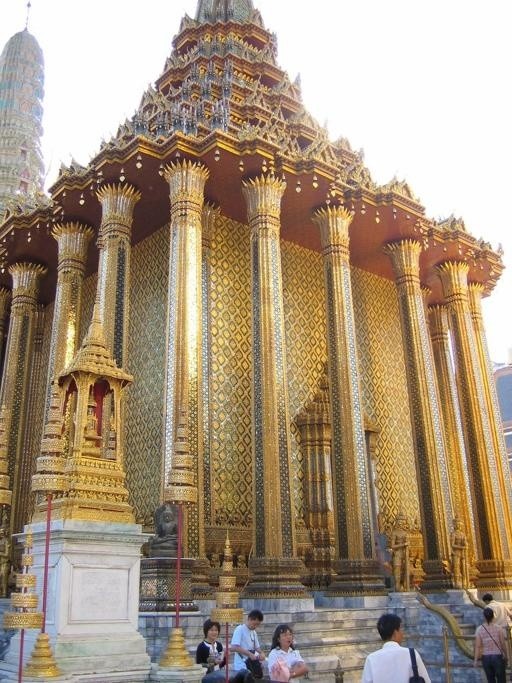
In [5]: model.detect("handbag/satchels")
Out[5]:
[246,656,263,678]
[502,655,508,664]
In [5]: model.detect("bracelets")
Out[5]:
[292,670,296,678]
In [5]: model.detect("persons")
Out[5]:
[361,614,433,683]
[229,609,265,671]
[196,618,227,673]
[474,606,508,683]
[149,507,179,548]
[0,525,12,598]
[480,593,512,639]
[391,514,411,592]
[267,623,308,683]
[449,514,471,588]
